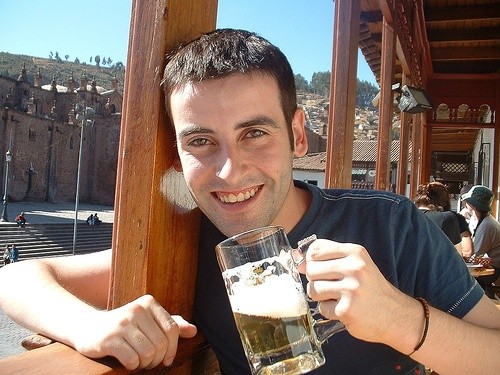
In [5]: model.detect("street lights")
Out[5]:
[0,148,12,223]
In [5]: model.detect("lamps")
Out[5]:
[398,84,437,114]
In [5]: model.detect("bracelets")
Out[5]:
[405,296,431,359]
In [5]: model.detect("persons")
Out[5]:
[93,212,102,225]
[413,183,462,258]
[458,185,500,289]
[0,28,500,375]
[390,183,397,193]
[15,212,26,228]
[3,244,10,265]
[85,214,94,226]
[9,244,19,263]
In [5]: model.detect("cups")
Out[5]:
[216,225,346,375]
